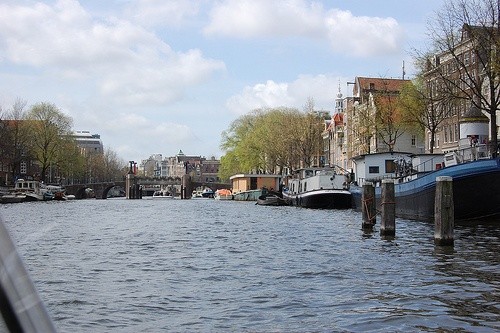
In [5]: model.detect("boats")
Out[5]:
[191,186,214,198]
[257,193,281,206]
[281,165,352,209]
[0,180,65,203]
[152,190,174,198]
[214,189,233,200]
